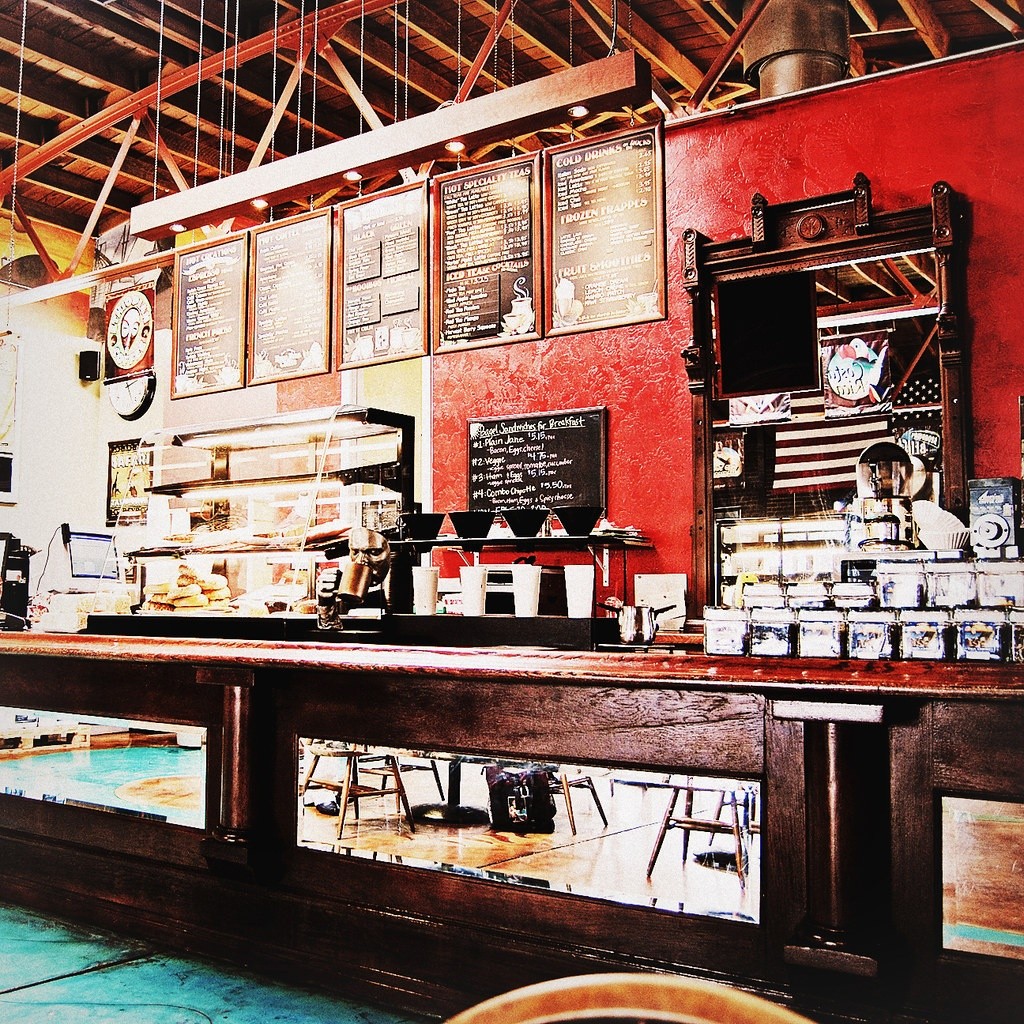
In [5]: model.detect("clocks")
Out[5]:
[107,373,155,420]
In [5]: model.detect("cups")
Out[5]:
[563,564,596,618]
[511,564,542,618]
[410,563,440,615]
[458,562,489,618]
[331,562,372,606]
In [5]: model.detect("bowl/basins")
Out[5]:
[553,505,609,537]
[401,512,444,539]
[448,509,495,539]
[501,508,550,537]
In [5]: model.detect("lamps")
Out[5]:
[126,0,653,242]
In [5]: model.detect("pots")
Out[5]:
[598,602,657,646]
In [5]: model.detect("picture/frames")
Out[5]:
[170,230,247,400]
[547,115,666,334]
[247,203,334,386]
[336,175,431,371]
[434,151,540,355]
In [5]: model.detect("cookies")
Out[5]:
[139,569,234,613]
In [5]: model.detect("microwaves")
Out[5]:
[478,562,570,619]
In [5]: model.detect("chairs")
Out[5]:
[300,738,760,896]
[438,967,810,1024]
